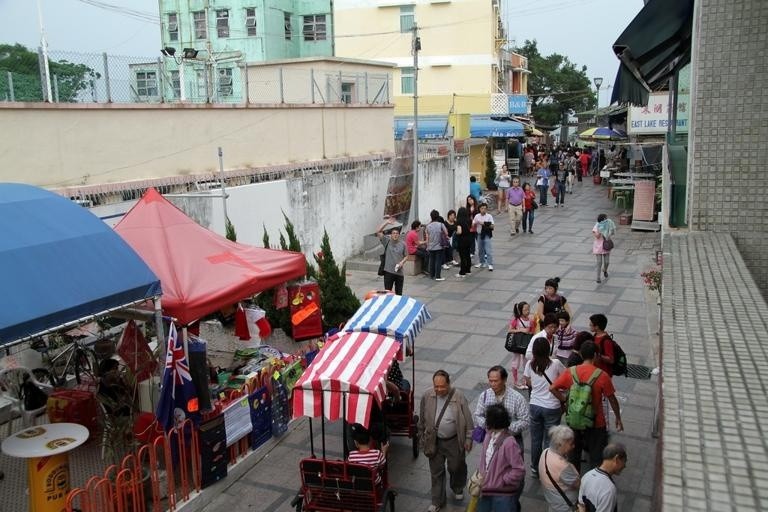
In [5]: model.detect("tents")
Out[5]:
[111,186,314,368]
[0,182,166,386]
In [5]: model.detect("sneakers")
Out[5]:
[474,263,487,268]
[603,271,609,278]
[467,272,472,276]
[451,489,463,499]
[524,384,530,389]
[435,278,445,281]
[455,273,465,278]
[597,280,602,284]
[451,260,458,265]
[428,503,440,511]
[514,382,523,389]
[488,264,494,270]
[530,469,538,477]
[442,263,450,270]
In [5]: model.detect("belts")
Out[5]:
[436,434,457,441]
[509,202,522,207]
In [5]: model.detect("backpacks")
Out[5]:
[565,366,602,432]
[594,332,628,378]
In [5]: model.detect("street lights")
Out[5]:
[593,77,603,175]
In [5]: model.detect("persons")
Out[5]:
[566,330,596,368]
[548,339,625,474]
[557,153,568,170]
[589,313,615,430]
[347,426,390,495]
[504,177,526,236]
[475,403,527,512]
[592,213,616,284]
[575,150,583,182]
[466,194,488,259]
[494,165,512,215]
[537,424,581,512]
[571,152,576,185]
[579,150,592,177]
[577,443,628,512]
[522,182,536,234]
[419,369,475,512]
[563,153,577,195]
[524,148,534,177]
[387,345,414,405]
[533,154,549,192]
[376,217,409,296]
[536,153,550,188]
[554,311,578,368]
[454,207,472,278]
[535,276,572,332]
[472,364,532,462]
[536,161,551,206]
[524,313,560,398]
[507,301,538,390]
[425,216,448,281]
[554,161,569,208]
[426,209,450,270]
[471,202,495,271]
[522,336,567,477]
[404,220,430,275]
[470,176,483,206]
[444,210,459,266]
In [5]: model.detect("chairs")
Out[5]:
[0,367,54,437]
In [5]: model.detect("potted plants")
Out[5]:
[94,338,163,504]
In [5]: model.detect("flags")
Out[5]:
[155,320,201,440]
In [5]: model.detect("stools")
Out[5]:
[614,196,626,210]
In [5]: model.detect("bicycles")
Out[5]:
[19,335,100,418]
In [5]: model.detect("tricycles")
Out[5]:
[341,293,432,457]
[290,330,403,512]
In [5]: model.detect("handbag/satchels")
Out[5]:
[471,390,487,443]
[377,240,392,276]
[551,184,556,198]
[532,198,537,210]
[504,316,534,354]
[467,432,512,497]
[439,223,451,249]
[494,179,499,187]
[602,233,615,252]
[423,387,458,458]
[532,293,547,334]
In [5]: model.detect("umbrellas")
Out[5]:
[575,126,624,175]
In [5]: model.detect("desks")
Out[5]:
[609,179,635,186]
[1,422,89,512]
[612,186,635,201]
[614,171,654,180]
